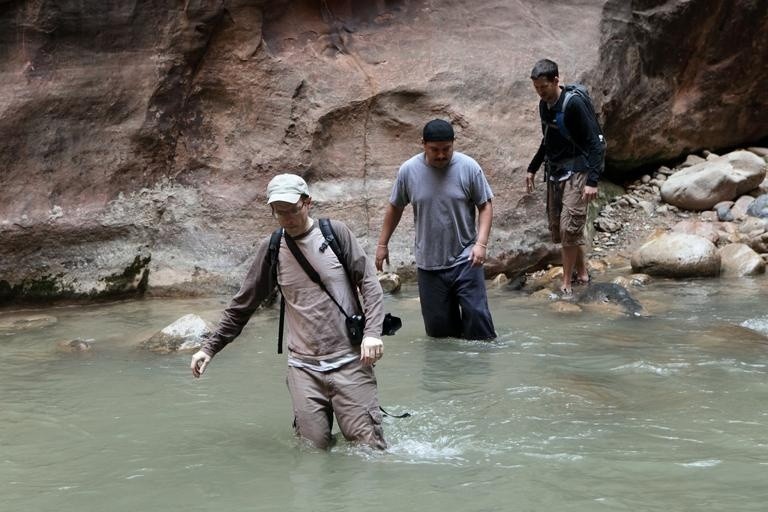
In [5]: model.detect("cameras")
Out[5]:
[346,313,401,346]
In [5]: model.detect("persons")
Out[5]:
[376,119,497,341]
[525,59,608,298]
[191,173,388,452]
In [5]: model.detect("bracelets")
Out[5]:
[377,244,387,248]
[476,242,489,249]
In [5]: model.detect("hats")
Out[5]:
[264,171,310,208]
[422,118,456,143]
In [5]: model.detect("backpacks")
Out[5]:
[539,83,607,175]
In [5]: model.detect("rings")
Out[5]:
[481,260,484,264]
